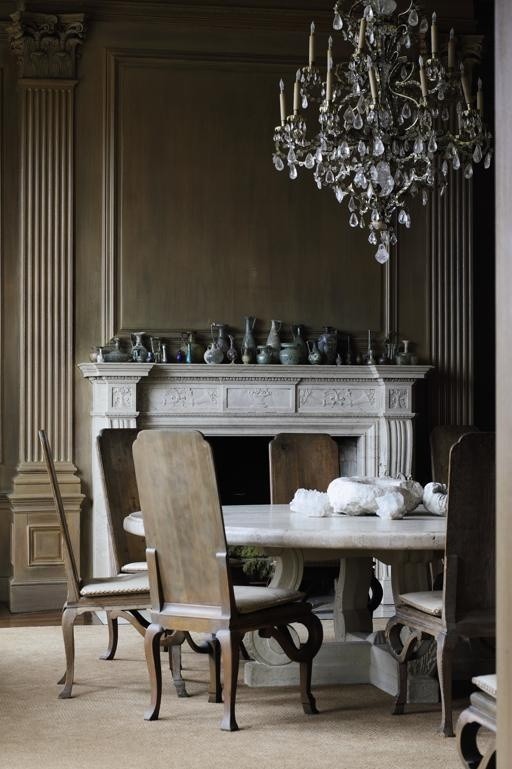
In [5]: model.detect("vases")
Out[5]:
[88,314,418,365]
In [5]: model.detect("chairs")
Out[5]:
[130,428,323,736]
[386,428,498,738]
[265,431,344,507]
[37,429,198,702]
[97,428,146,662]
[456,670,498,769]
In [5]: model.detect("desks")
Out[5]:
[119,493,447,703]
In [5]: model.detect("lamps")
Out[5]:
[271,1,495,265]
